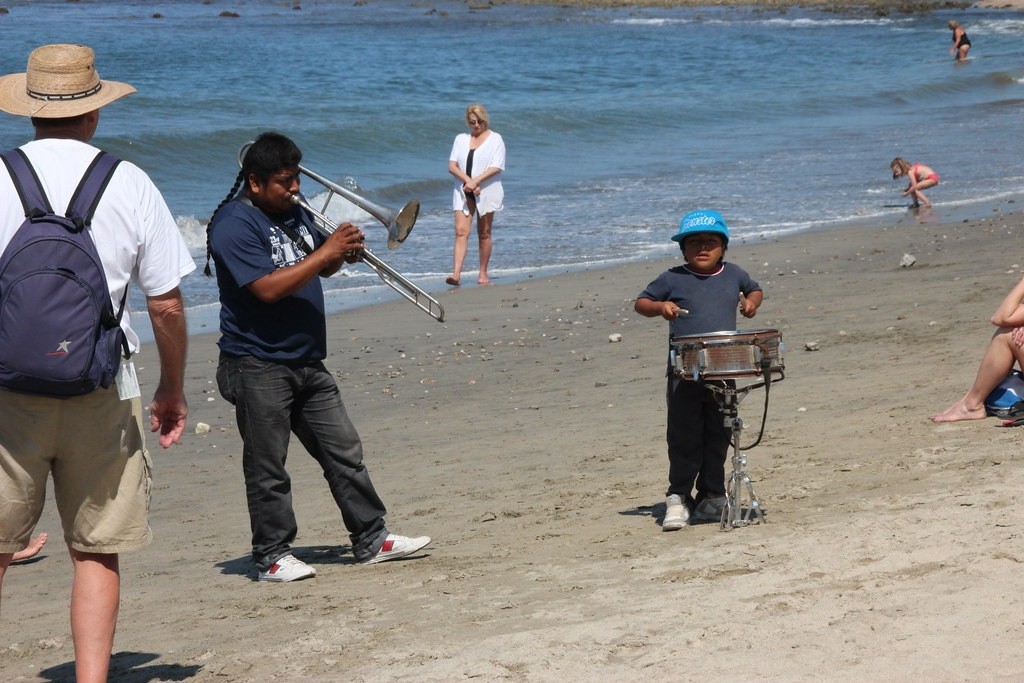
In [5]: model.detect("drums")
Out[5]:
[669,328,786,382]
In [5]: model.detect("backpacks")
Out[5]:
[0,148,130,399]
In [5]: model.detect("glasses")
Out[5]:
[470,118,483,125]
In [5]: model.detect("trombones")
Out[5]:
[237,140,445,323]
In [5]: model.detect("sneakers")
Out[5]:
[364,532,432,564]
[695,493,728,521]
[257,553,317,583]
[662,494,691,530]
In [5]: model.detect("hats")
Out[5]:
[0,44,139,119]
[670,210,730,244]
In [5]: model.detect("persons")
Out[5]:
[8,531,48,560]
[929,276,1024,428]
[634,211,763,530]
[890,158,939,208]
[947,20,972,60]
[0,42,199,682]
[445,104,507,286]
[204,130,434,584]
